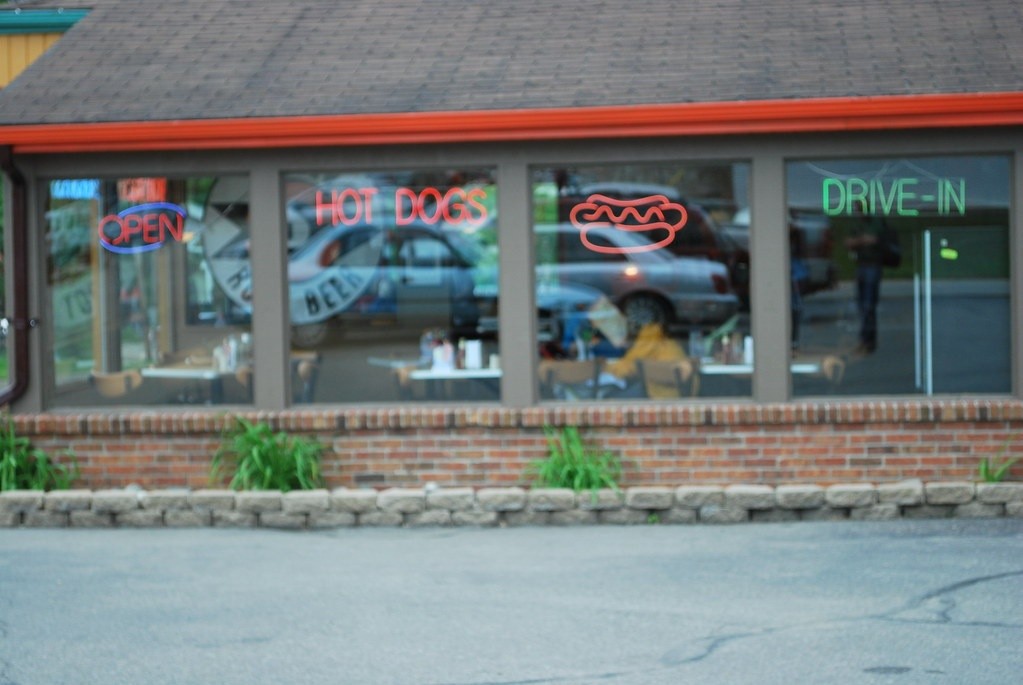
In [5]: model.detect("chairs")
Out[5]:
[89,347,321,406]
[389,323,862,403]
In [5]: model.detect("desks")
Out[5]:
[140,362,241,406]
[411,368,505,404]
[699,356,822,398]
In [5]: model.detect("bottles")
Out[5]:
[714,330,753,365]
[419,328,467,371]
[213,331,249,372]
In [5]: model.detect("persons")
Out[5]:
[840,216,906,353]
[417,324,695,400]
[786,209,808,351]
[445,257,482,340]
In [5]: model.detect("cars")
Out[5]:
[284,184,836,349]
